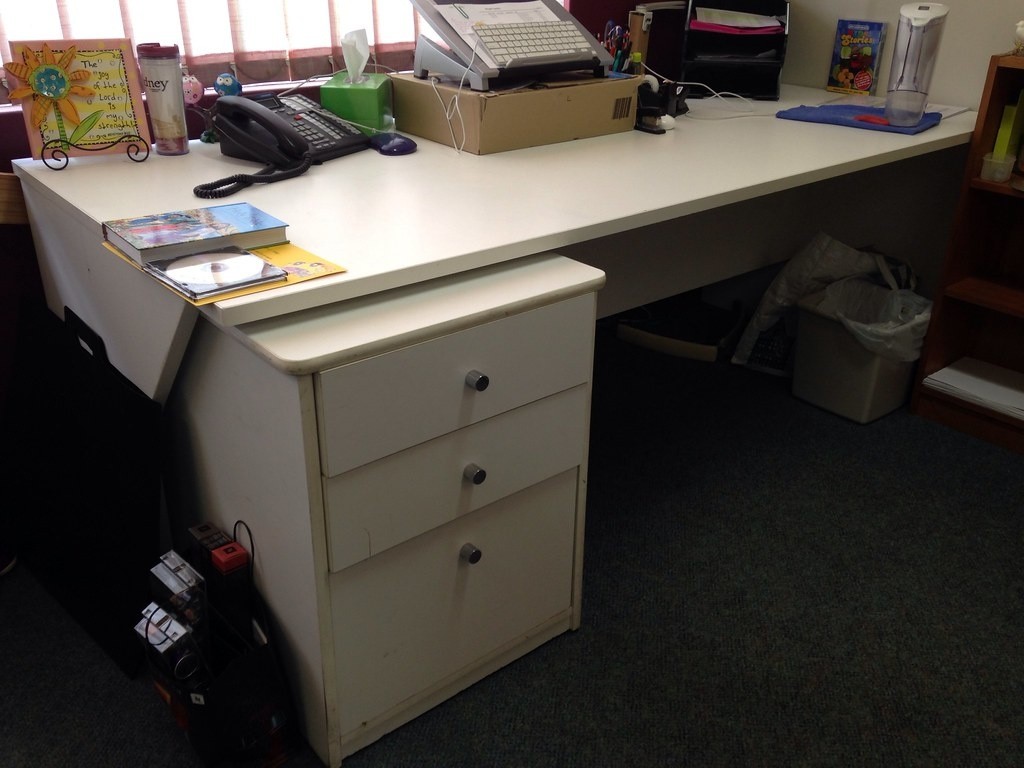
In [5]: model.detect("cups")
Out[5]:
[136,42,190,156]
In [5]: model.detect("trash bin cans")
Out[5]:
[791,277,934,424]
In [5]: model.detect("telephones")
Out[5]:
[214,92,372,172]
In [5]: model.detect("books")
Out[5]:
[101,203,291,268]
[825,19,887,97]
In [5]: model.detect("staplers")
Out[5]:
[633,107,667,135]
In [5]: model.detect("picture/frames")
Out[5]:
[2,38,152,170]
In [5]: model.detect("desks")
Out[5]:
[10,80,980,404]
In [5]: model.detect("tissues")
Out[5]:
[319,29,393,138]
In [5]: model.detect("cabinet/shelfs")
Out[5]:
[176,252,605,768]
[679,0,792,102]
[907,51,1024,454]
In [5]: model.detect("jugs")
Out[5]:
[883,3,950,127]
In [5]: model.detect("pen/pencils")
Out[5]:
[597,20,633,73]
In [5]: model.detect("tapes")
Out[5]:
[637,75,659,92]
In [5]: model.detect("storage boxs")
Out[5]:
[387,73,638,157]
